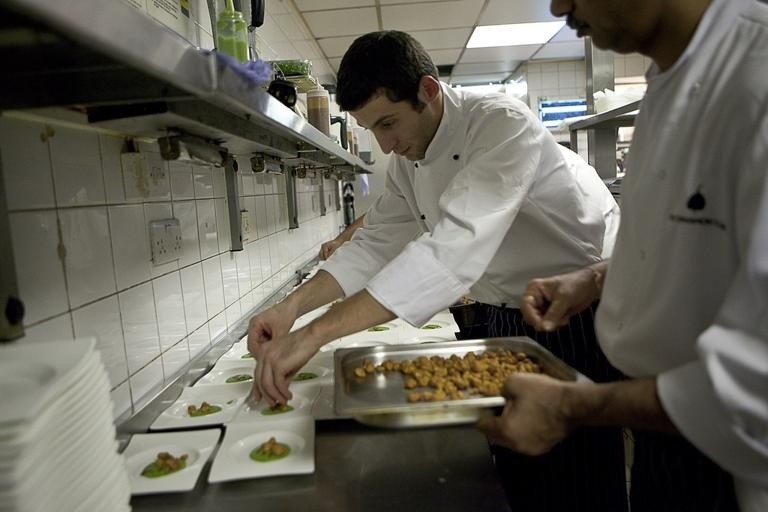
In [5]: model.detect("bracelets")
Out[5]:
[581,262,605,296]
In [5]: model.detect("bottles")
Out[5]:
[343,194,355,225]
[216,0,249,61]
[307,77,331,137]
[353,123,359,155]
[346,117,354,154]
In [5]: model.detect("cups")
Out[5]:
[208,0,265,62]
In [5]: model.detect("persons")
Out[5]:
[478,0,768,512]
[247,31,621,511]
[318,206,371,261]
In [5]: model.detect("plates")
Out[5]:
[211,419,315,484]
[121,429,222,495]
[0,335,132,512]
[194,360,256,385]
[289,364,325,382]
[229,375,321,426]
[313,379,351,422]
[148,385,254,429]
[219,259,459,358]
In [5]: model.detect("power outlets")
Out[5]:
[149,218,187,267]
[239,210,251,242]
